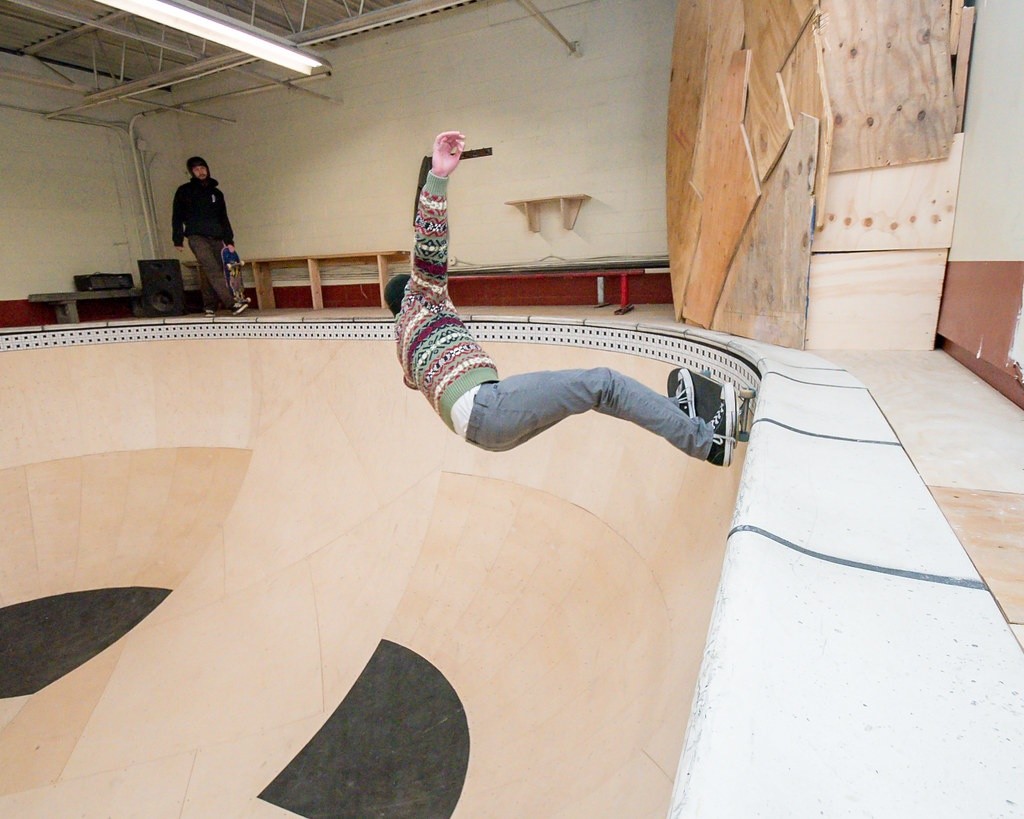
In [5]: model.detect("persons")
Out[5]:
[172,154,250,317]
[383,130,738,470]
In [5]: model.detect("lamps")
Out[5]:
[94,0,333,77]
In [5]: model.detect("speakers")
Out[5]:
[137,258,189,318]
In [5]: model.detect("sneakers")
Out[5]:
[204,309,217,317]
[232,303,248,316]
[676,368,696,419]
[705,382,738,467]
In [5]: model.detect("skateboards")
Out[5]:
[222,247,252,304]
[667,367,755,448]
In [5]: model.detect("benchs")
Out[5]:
[28,286,145,325]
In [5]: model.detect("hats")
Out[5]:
[383,273,412,308]
